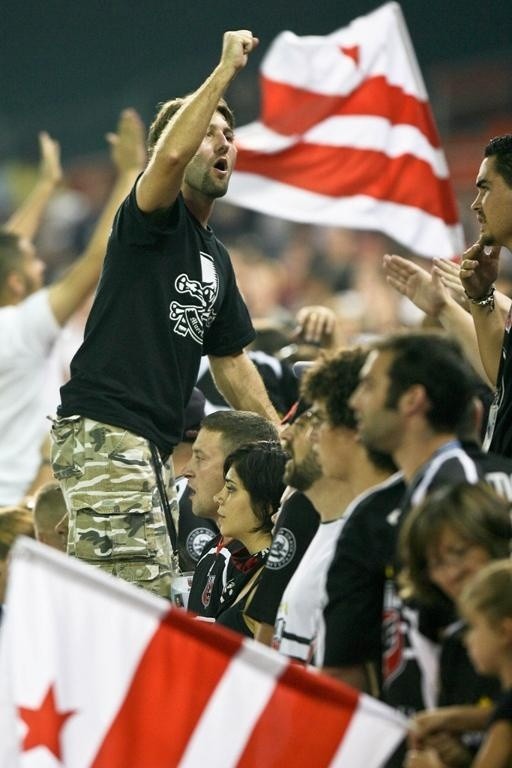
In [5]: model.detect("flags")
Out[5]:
[213,1,467,262]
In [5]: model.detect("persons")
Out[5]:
[47,27,289,605]
[459,133,512,457]
[0,108,146,603]
[170,224,512,767]
